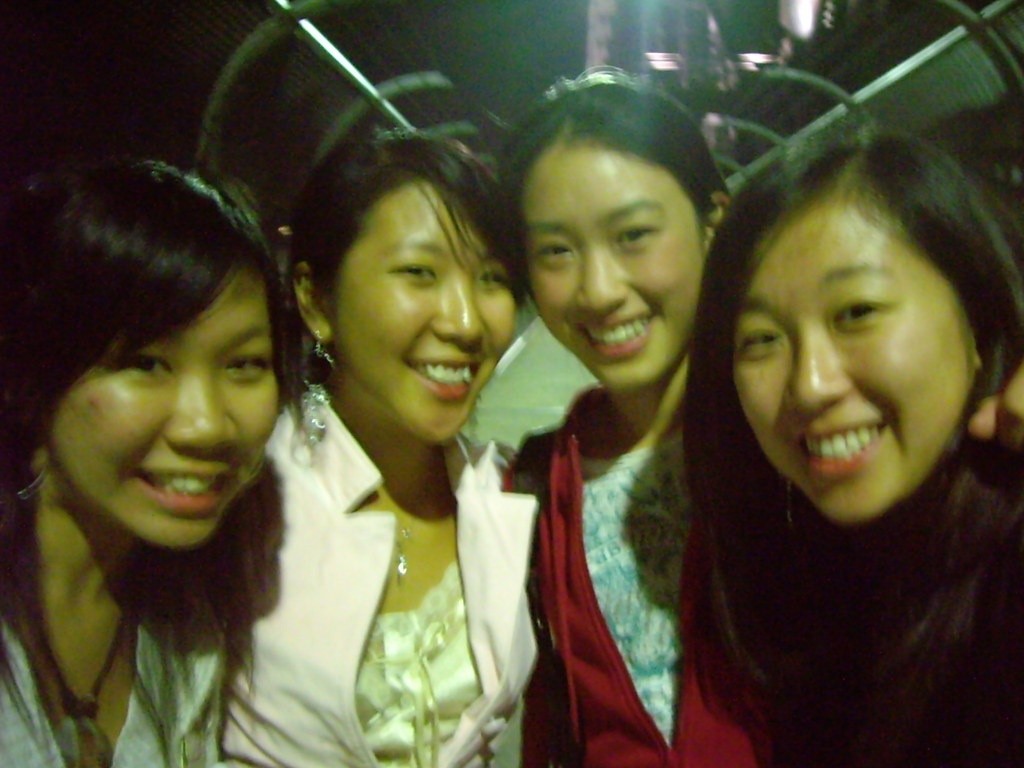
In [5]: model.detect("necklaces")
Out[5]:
[30,614,126,768]
[394,527,412,578]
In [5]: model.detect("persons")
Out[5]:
[0,157,283,768]
[681,134,1024,768]
[496,80,1024,768]
[218,133,538,768]
